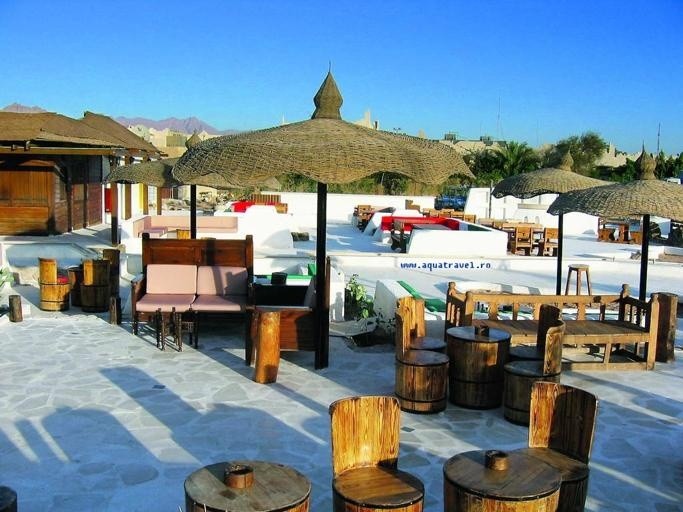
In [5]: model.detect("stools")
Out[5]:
[565,264,592,308]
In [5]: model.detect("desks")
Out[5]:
[184,461,312,512]
[443,449,562,512]
[447,323,512,410]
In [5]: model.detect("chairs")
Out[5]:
[37,249,120,312]
[510,304,562,361]
[353,199,558,257]
[521,380,599,512]
[395,308,450,415]
[329,394,425,512]
[396,296,447,354]
[597,216,639,244]
[244,255,330,370]
[503,319,566,427]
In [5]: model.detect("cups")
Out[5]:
[224,465,254,488]
[475,325,489,336]
[485,450,509,471]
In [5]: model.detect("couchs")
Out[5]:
[249,194,287,213]
[132,215,238,239]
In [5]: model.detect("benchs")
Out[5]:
[130,233,254,334]
[444,281,660,370]
